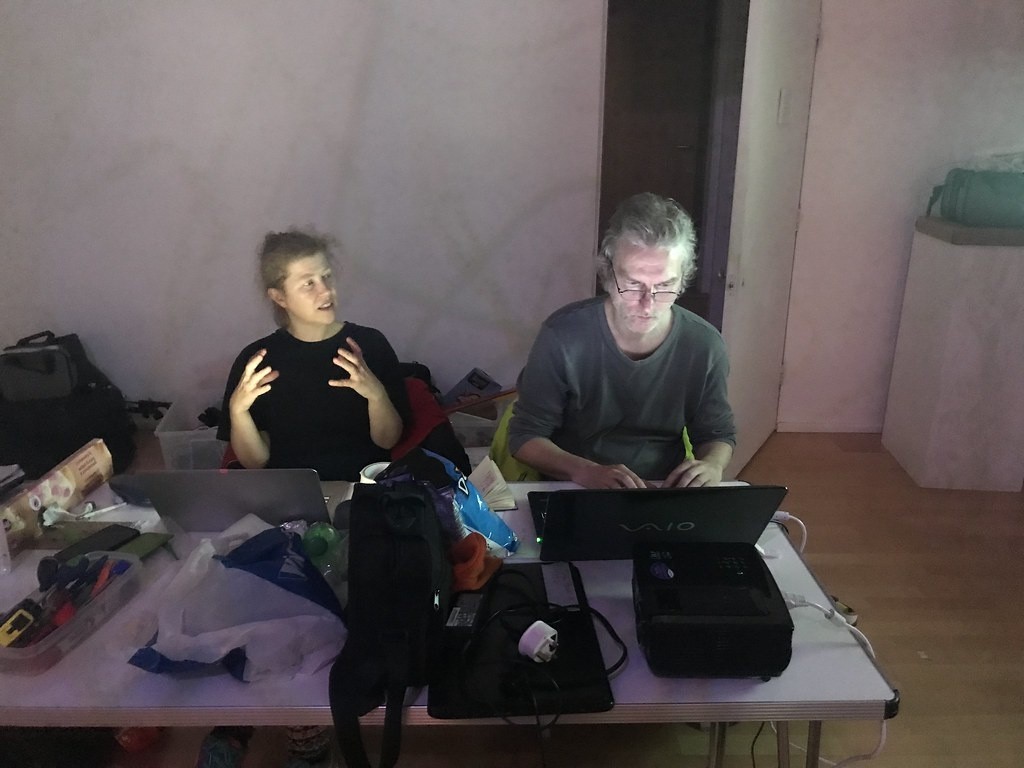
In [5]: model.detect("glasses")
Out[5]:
[607,257,684,302]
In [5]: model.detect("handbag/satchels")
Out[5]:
[926,168,1024,227]
[0,331,132,480]
[127,526,349,684]
[344,449,464,687]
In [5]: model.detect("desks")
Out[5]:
[0,480,900,768]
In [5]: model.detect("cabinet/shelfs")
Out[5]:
[881,215,1024,493]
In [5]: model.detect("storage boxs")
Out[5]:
[442,385,518,447]
[0,550,141,676]
[155,394,229,470]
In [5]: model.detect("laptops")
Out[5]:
[136,467,348,533]
[427,560,615,719]
[526,485,788,561]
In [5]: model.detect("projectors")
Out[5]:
[632,543,794,682]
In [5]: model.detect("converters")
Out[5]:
[441,589,491,643]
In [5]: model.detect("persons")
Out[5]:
[507,192,738,487]
[216,230,407,480]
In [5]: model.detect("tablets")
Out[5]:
[54,524,139,562]
